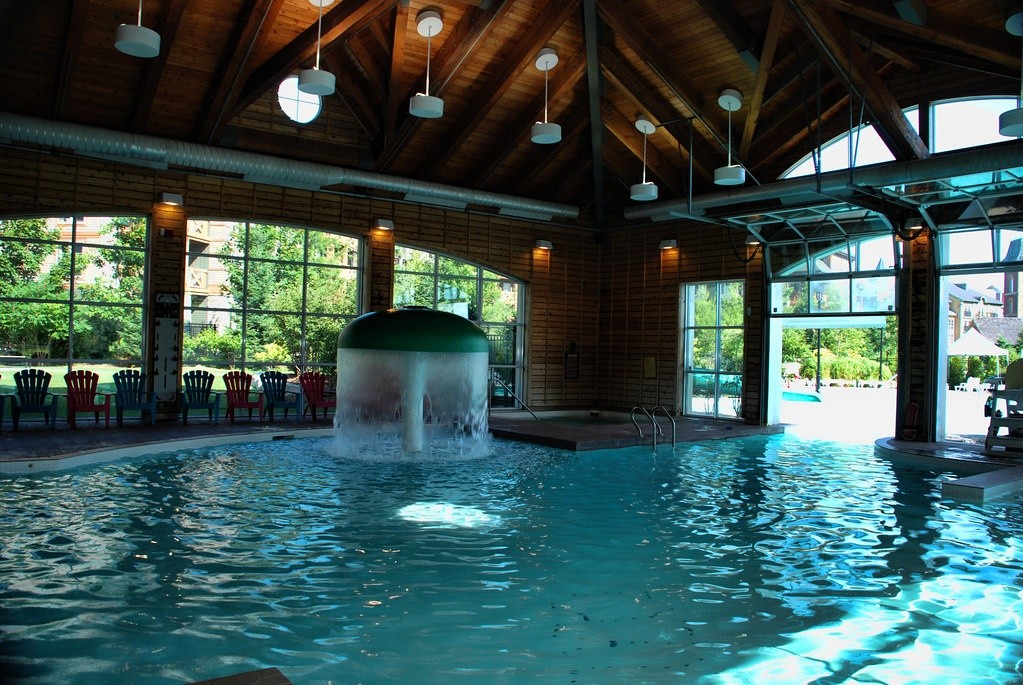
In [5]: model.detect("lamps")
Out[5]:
[409,11,448,117]
[535,240,553,249]
[744,235,759,245]
[630,114,658,201]
[659,240,676,249]
[904,218,923,229]
[532,47,562,144]
[713,89,745,185]
[115,0,160,58]
[298,0,335,95]
[159,193,183,206]
[374,219,394,230]
[998,12,1023,136]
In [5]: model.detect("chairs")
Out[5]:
[984,358,1023,459]
[260,371,303,425]
[180,370,222,426]
[110,369,159,425]
[954,377,981,392]
[63,370,113,428]
[804,378,889,388]
[220,371,265,425]
[299,371,336,424]
[8,369,60,430]
